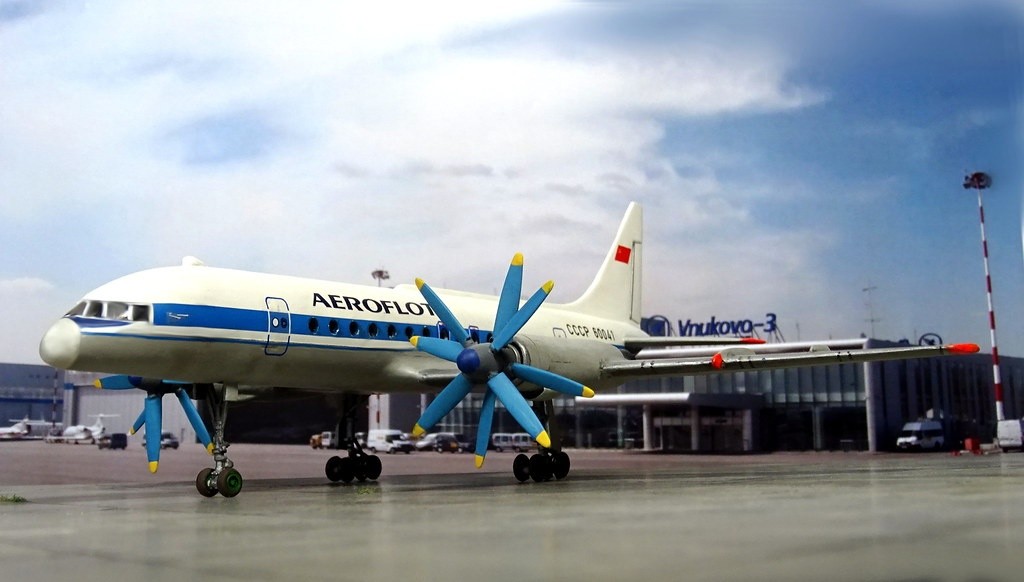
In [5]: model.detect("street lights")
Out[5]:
[963,171,1008,420]
[370,268,390,289]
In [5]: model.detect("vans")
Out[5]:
[997,419,1024,452]
[896,421,944,449]
[492,432,534,452]
[368,429,414,454]
[142,430,179,450]
[310,431,367,449]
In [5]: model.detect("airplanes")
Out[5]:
[39,201,981,498]
[0,413,121,444]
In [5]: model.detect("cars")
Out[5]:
[416,432,475,454]
[98,433,127,450]
